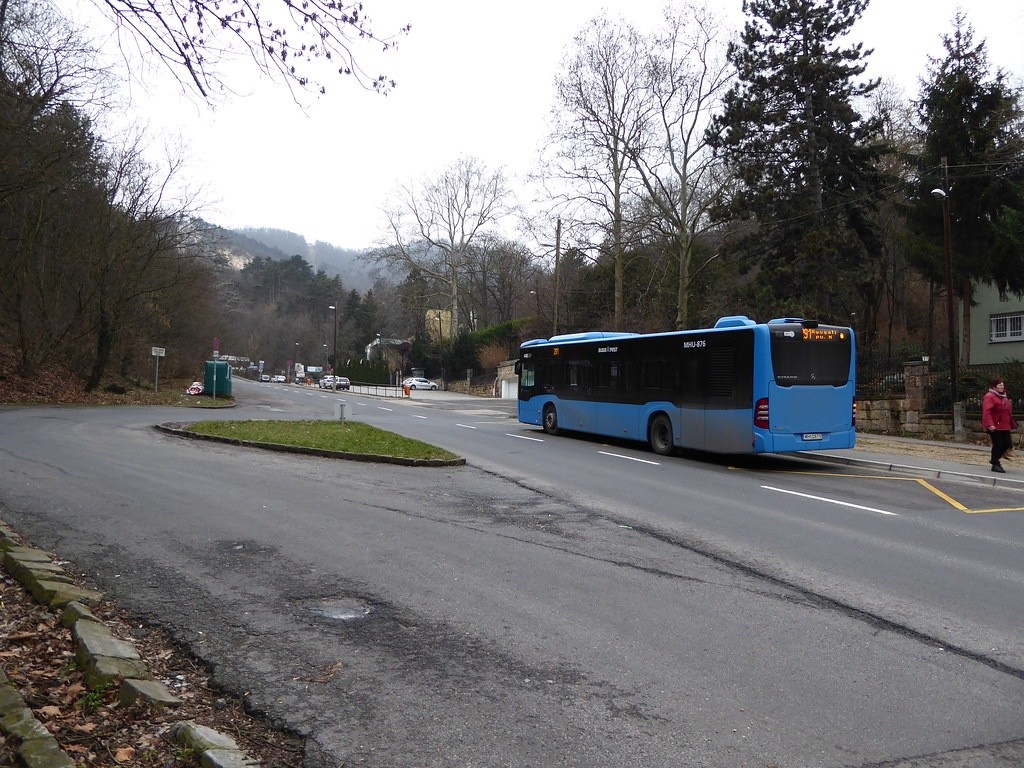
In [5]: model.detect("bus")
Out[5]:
[515,316,857,455]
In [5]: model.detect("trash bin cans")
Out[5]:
[405,384,410,396]
[204,361,232,394]
[295,377,300,384]
[308,379,311,385]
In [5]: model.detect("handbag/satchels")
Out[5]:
[1010,416,1019,430]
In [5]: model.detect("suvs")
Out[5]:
[319,376,339,388]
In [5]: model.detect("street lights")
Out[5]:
[931,187,958,401]
[328,301,337,376]
[529,291,558,337]
[435,312,443,358]
[377,331,381,354]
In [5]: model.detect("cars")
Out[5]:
[261,375,269,382]
[331,378,350,390]
[274,376,286,383]
[402,377,439,390]
[271,375,281,382]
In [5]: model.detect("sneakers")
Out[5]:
[989,460,1001,467]
[991,466,1006,473]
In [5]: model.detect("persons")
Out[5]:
[982,378,1013,473]
